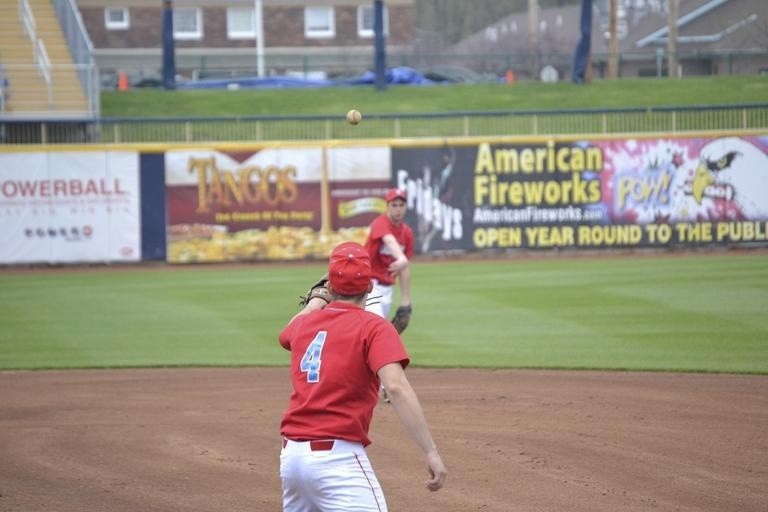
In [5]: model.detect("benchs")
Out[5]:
[1,0,102,119]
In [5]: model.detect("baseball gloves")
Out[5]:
[300,273,332,303]
[392,306,410,333]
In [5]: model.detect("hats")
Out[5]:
[328,241,372,295]
[384,187,407,204]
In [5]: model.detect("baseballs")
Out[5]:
[347,109,363,126]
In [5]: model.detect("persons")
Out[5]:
[357,187,415,405]
[276,240,449,511]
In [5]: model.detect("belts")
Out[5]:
[280,437,336,452]
[375,278,391,286]
